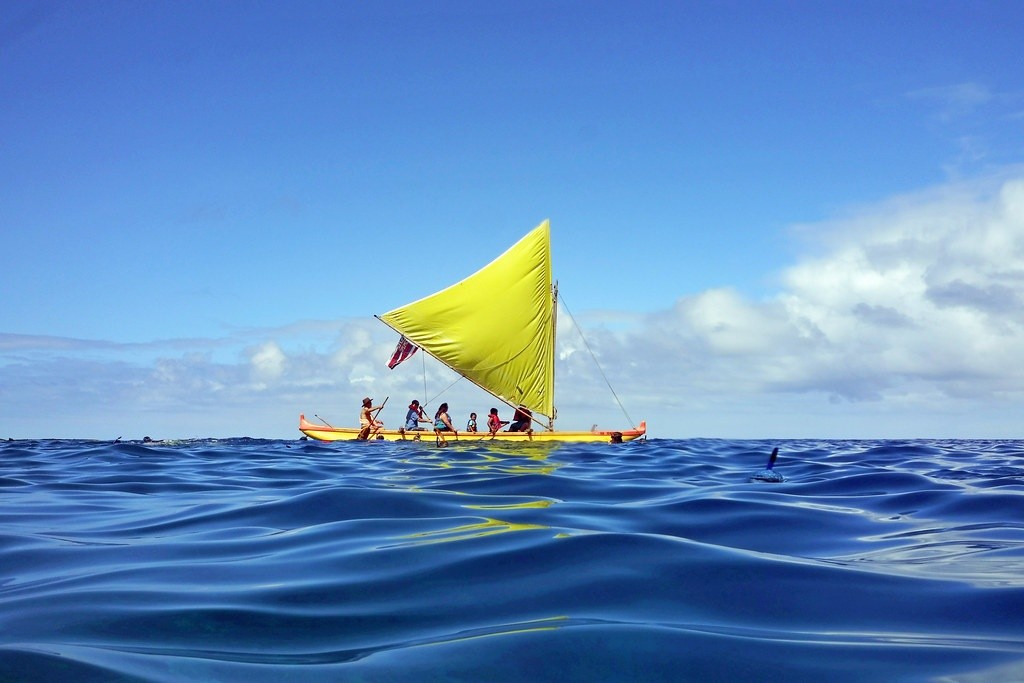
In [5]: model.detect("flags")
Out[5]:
[386,336,419,370]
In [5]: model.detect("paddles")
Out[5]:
[476,422,508,442]
[420,405,445,441]
[357,396,390,440]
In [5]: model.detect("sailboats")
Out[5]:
[298,217,646,444]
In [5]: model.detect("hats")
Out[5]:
[362,397,373,407]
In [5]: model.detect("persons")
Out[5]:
[405,400,432,431]
[505,406,530,432]
[468,413,477,433]
[435,403,456,431]
[360,398,383,431]
[488,408,510,433]
